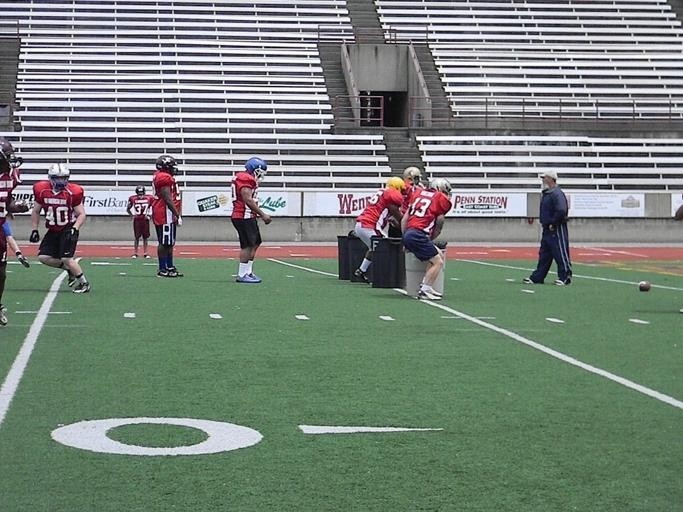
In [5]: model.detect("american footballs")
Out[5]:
[639,280,650,290]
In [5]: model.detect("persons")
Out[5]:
[30,163,91,294]
[388,167,426,237]
[152,154,183,277]
[353,177,406,285]
[0,137,31,326]
[127,186,156,259]
[231,157,272,282]
[401,178,452,300]
[523,171,572,286]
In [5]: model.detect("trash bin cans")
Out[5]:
[337,230,448,296]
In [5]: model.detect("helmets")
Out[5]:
[156,155,178,177]
[0,137,14,154]
[49,163,70,189]
[245,157,268,182]
[385,166,452,197]
[136,185,145,194]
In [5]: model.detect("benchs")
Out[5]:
[372,0,683,196]
[0,1,396,194]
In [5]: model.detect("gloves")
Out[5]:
[65,227,78,240]
[29,230,39,243]
[177,215,183,226]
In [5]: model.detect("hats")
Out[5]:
[539,169,558,182]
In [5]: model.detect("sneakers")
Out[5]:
[156,269,183,278]
[131,254,136,258]
[354,268,370,284]
[236,272,263,283]
[144,255,150,259]
[68,269,90,294]
[18,254,30,269]
[523,277,533,284]
[554,279,565,285]
[418,282,443,300]
